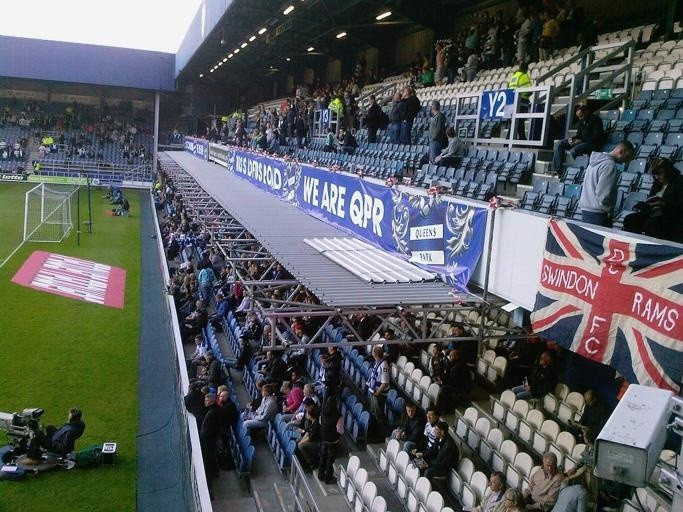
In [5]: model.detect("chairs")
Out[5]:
[0,95,158,183]
[155,19,683,512]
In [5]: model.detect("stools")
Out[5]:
[98,440,119,467]
